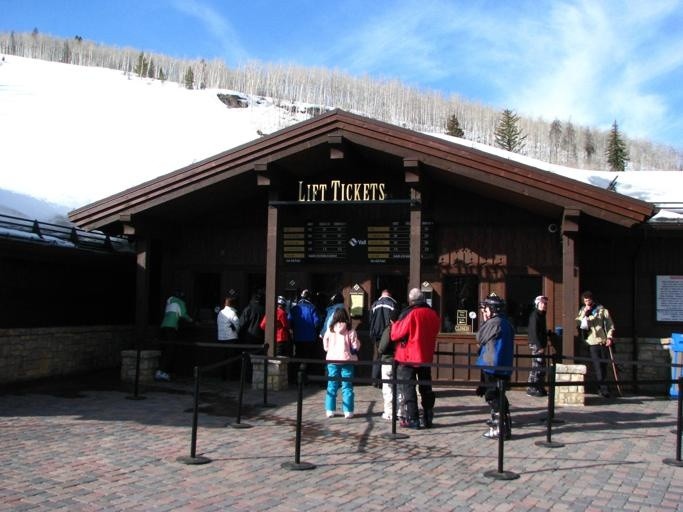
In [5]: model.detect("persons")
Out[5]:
[527,296,551,396]
[217,289,345,388]
[152,287,194,380]
[390,286,441,428]
[475,296,514,440]
[323,308,358,419]
[579,288,617,397]
[369,290,400,387]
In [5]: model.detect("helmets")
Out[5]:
[479,292,507,313]
[327,292,344,304]
[534,296,550,308]
[299,289,311,299]
[276,295,288,306]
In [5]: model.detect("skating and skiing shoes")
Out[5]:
[481,410,511,439]
[525,371,548,397]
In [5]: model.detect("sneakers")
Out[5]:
[399,414,434,429]
[326,410,353,418]
[153,368,172,383]
[595,381,612,400]
